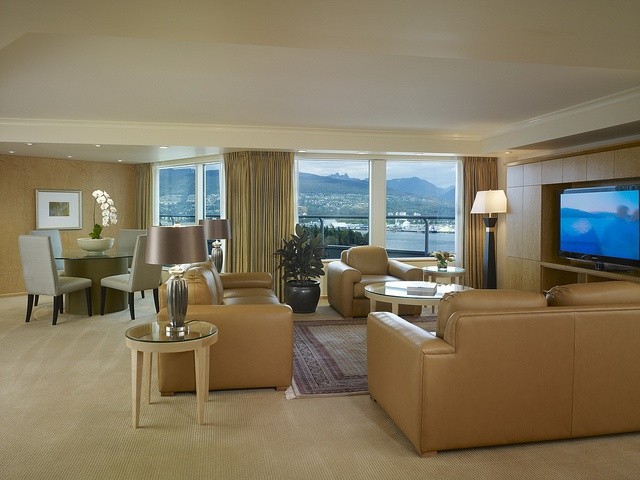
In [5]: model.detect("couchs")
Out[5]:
[327,245,423,319]
[367,280,640,458]
[157,259,294,396]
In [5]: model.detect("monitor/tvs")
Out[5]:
[557,183,640,270]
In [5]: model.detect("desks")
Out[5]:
[422,266,466,313]
[54,250,133,314]
[365,281,475,316]
[126,320,218,429]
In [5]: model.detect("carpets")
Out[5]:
[294,316,437,394]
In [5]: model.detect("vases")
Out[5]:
[77,238,114,256]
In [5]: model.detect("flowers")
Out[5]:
[86,189,117,239]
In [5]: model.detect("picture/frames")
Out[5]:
[35,188,82,230]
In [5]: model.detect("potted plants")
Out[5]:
[273,223,329,313]
[429,251,455,271]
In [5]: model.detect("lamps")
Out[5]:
[145,223,209,332]
[469,188,507,288]
[199,217,232,274]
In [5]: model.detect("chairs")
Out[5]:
[100,235,159,321]
[29,229,65,306]
[19,235,92,325]
[115,229,147,299]
